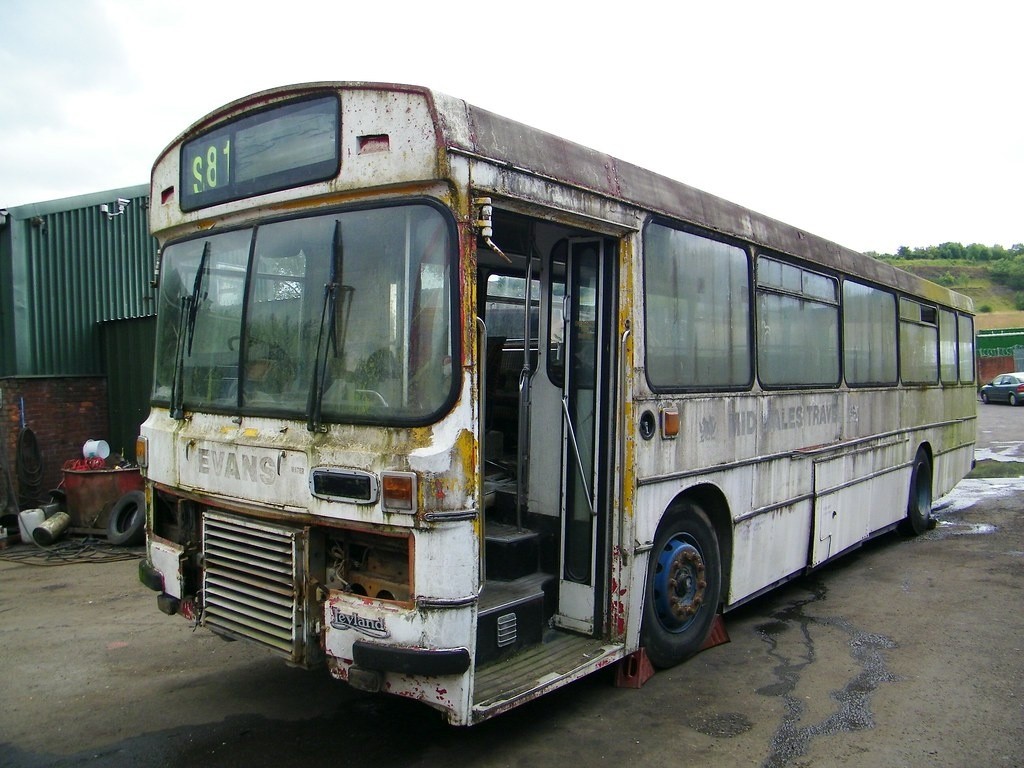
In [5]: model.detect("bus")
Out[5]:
[136,81,978,727]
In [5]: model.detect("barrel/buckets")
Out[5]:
[82,438,110,459]
[61,466,141,529]
[34,511,71,546]
[17,508,45,544]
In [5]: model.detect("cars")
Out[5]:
[979,372,1024,406]
